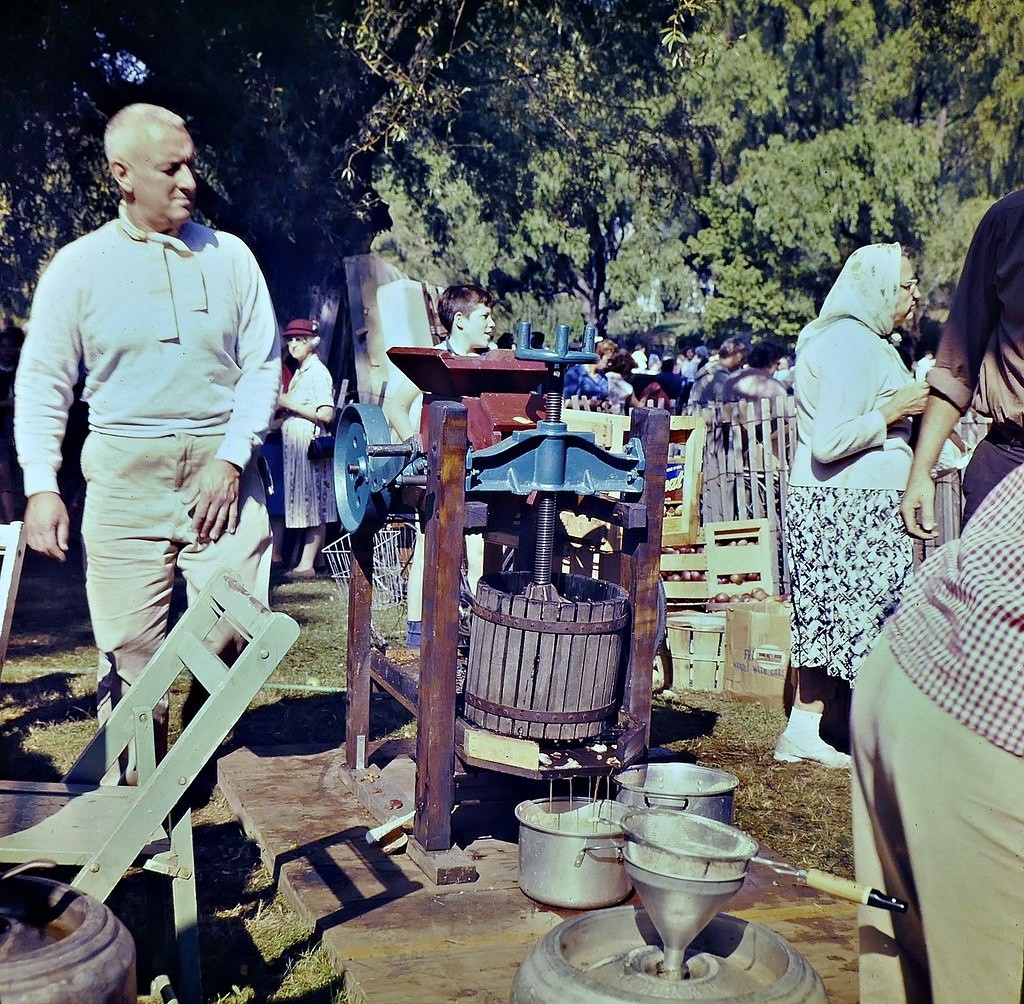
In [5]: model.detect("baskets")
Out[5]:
[321,523,402,610]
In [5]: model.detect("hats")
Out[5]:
[284,318,316,337]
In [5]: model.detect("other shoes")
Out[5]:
[283,567,315,579]
[774,733,851,770]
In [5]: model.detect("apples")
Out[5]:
[658,539,792,606]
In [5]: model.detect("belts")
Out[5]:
[992,421,1024,448]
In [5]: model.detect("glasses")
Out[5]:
[900,279,917,295]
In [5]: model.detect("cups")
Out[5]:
[916,367,933,381]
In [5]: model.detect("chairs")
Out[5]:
[0,521,300,1004]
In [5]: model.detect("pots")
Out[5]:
[515,796,633,909]
[612,762,740,826]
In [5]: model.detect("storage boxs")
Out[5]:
[560,408,779,613]
[723,602,793,712]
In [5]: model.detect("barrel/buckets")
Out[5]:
[509,907,829,1004]
[0,875,136,1004]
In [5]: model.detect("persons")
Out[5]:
[277,319,336,579]
[773,241,967,770]
[387,286,500,649]
[898,189,1023,541]
[497,322,796,455]
[848,463,1022,1002]
[911,350,936,382]
[15,103,284,786]
